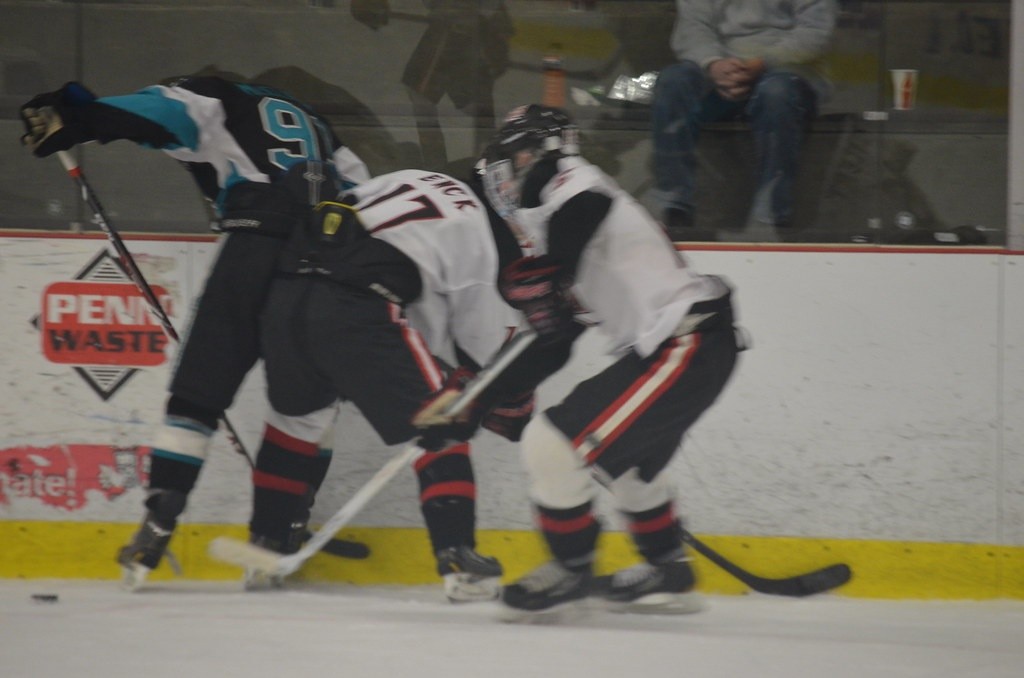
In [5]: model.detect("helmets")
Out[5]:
[483,103,578,220]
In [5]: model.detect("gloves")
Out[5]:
[20,84,93,156]
[413,367,491,452]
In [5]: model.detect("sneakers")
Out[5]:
[600,550,700,615]
[435,545,502,603]
[504,559,599,623]
[241,531,303,591]
[115,511,171,592]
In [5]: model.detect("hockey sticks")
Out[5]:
[586,473,854,600]
[37,103,375,564]
[206,328,538,578]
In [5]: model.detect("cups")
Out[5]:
[890,69,919,110]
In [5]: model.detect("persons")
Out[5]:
[484,122,739,627]
[650,0,839,242]
[21,62,357,591]
[246,167,532,602]
[341,1,517,165]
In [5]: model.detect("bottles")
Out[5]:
[542,57,568,110]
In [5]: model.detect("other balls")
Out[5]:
[30,593,58,603]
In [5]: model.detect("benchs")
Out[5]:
[311,102,1009,136]
